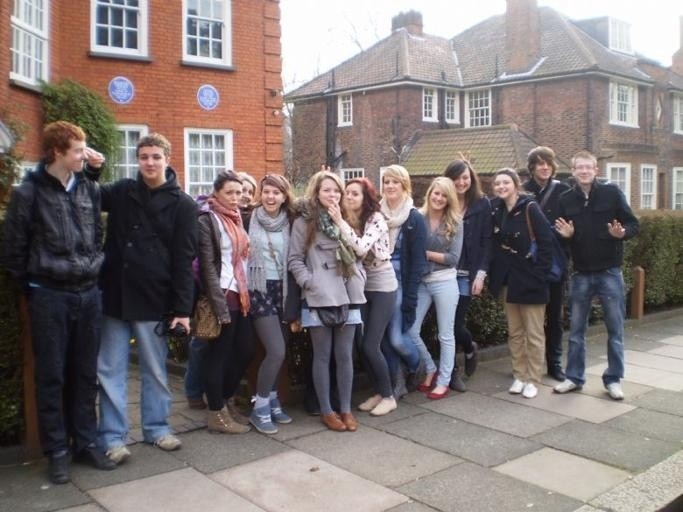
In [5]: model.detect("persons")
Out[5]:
[183,168,260,412]
[0,119,119,485]
[199,170,253,436]
[328,178,398,418]
[520,146,573,384]
[376,163,428,405]
[439,159,494,395]
[240,166,305,435]
[405,176,466,401]
[76,129,202,471]
[550,149,641,402]
[487,168,555,398]
[287,169,369,432]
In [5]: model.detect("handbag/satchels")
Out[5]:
[283,327,308,373]
[526,201,567,284]
[192,296,223,340]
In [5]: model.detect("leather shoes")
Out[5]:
[359,392,383,410]
[451,370,466,391]
[428,384,450,399]
[320,411,346,431]
[548,368,566,381]
[418,371,437,391]
[370,396,398,415]
[340,412,357,431]
[464,340,478,375]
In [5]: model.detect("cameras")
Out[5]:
[158,308,187,341]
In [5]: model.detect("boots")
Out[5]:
[207,407,252,434]
[224,395,250,426]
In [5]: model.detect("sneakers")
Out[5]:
[553,379,583,394]
[270,391,292,423]
[509,379,527,394]
[522,382,538,398]
[73,443,117,469]
[50,448,70,484]
[607,382,624,400]
[151,431,180,451]
[104,440,131,464]
[248,395,278,434]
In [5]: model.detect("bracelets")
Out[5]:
[476,270,488,277]
